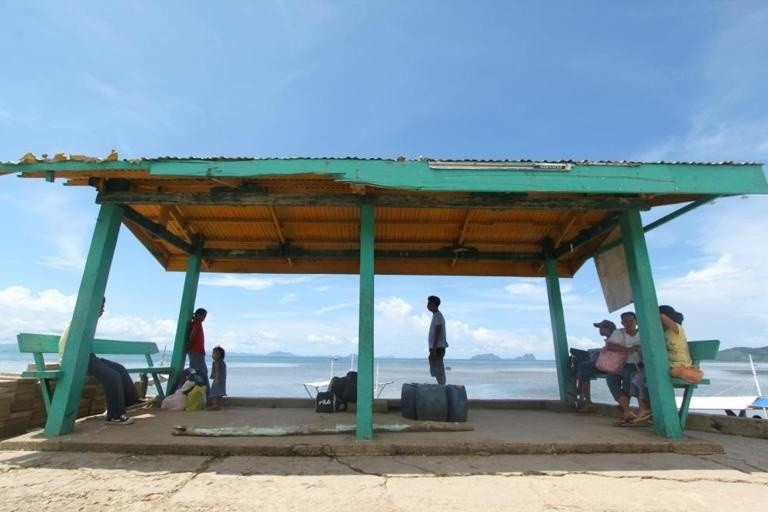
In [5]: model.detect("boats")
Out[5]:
[674,353,767,421]
[303,355,395,398]
[134,345,168,387]
[444,366,452,370]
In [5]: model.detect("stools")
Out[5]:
[302,380,329,397]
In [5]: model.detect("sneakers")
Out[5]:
[125,400,148,410]
[104,413,134,425]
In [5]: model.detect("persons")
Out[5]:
[57,298,146,425]
[628,305,693,425]
[187,308,207,389]
[606,311,639,423]
[425,294,447,386]
[565,320,615,413]
[205,346,227,411]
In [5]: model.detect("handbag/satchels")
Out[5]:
[595,350,626,374]
[315,372,357,413]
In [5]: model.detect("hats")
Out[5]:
[593,320,616,330]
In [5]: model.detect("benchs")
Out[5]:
[572,338,722,432]
[13,330,174,417]
